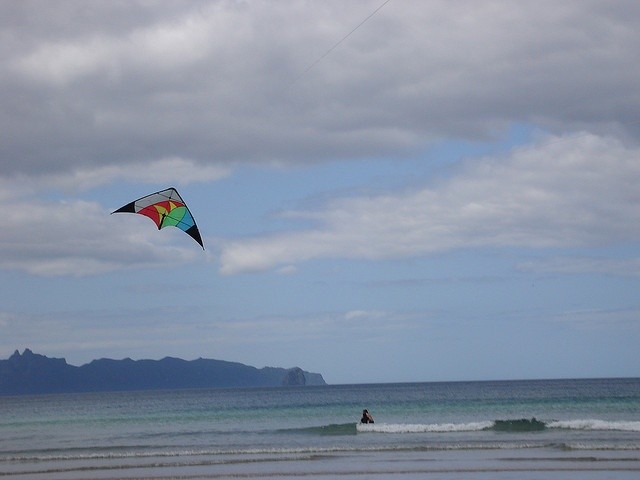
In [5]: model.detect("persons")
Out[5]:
[364,409,374,422]
[362,414,369,423]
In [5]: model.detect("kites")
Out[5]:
[111,188,205,249]
